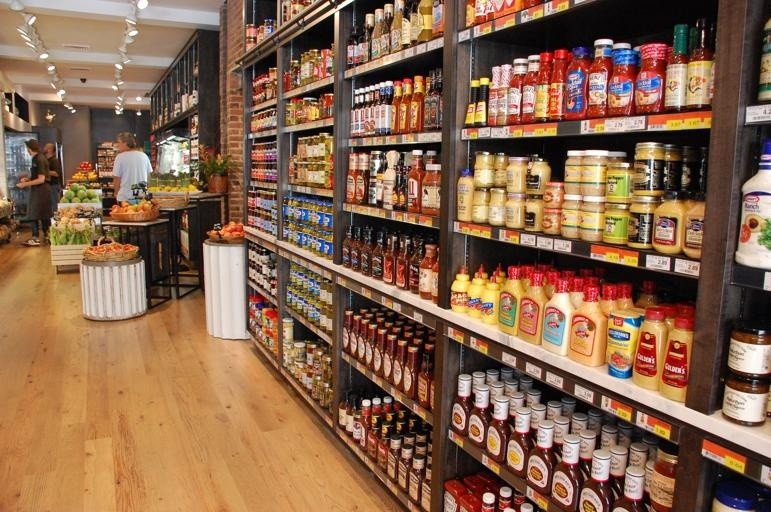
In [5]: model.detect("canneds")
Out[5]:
[369,151,383,177]
[726,317,771,382]
[245,19,277,53]
[294,132,333,189]
[368,178,376,205]
[282,318,333,417]
[472,141,708,258]
[722,368,771,426]
[285,93,334,126]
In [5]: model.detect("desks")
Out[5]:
[101,217,170,309]
[183,191,227,293]
[158,205,200,299]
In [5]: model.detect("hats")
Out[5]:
[24,139,40,152]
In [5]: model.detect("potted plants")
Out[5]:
[192,145,235,192]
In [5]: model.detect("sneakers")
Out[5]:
[20,239,41,246]
[43,237,50,248]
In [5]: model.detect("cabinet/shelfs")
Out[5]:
[148,29,219,153]
[235,0,770,512]
[95,143,117,207]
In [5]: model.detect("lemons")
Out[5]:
[148,184,198,193]
[72,171,97,180]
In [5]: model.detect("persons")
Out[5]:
[42,143,63,218]
[15,139,54,246]
[111,131,153,205]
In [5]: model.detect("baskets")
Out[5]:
[83,236,140,262]
[206,230,244,244]
[149,192,190,209]
[109,202,160,222]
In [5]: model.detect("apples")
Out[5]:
[112,200,153,213]
[211,221,244,238]
[60,183,100,203]
[80,160,93,171]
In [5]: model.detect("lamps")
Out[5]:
[112,0,148,116]
[9,0,77,115]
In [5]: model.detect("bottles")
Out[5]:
[407,149,443,215]
[340,302,434,416]
[447,261,695,405]
[450,365,678,512]
[336,387,433,512]
[730,141,771,273]
[340,223,437,304]
[348,68,443,136]
[344,0,444,67]
[461,19,716,126]
